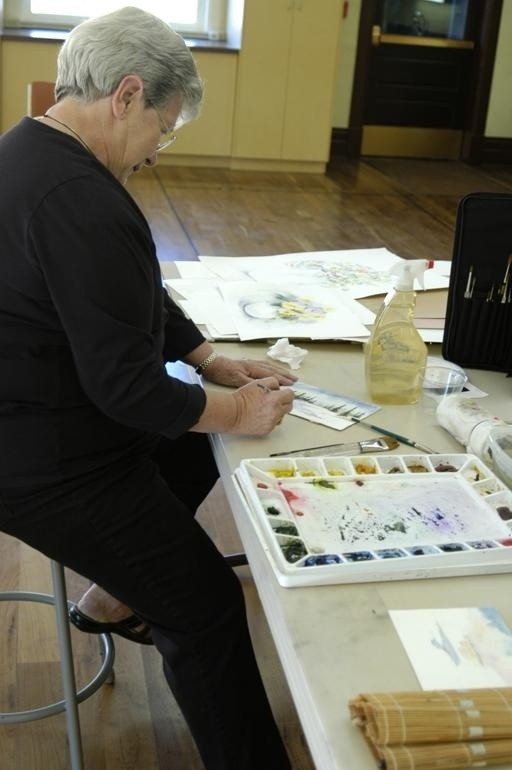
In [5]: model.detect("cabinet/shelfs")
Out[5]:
[0,26,239,171]
[234,0,347,173]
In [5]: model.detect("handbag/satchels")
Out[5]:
[441,191,511,373]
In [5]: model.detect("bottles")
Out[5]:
[436,392,510,460]
[411,11,426,34]
[449,0,467,38]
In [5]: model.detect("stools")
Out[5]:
[0,534,114,770]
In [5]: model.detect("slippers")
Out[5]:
[70,604,155,646]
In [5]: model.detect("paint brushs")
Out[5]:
[352,418,433,454]
[270,436,400,457]
[464,254,512,304]
[257,383,272,392]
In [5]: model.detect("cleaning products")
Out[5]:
[365,259,434,404]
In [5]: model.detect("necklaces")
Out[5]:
[41,113,94,157]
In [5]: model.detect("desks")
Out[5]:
[159,259,511,769]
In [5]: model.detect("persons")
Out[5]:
[0,5,292,770]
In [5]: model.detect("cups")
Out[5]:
[418,366,467,417]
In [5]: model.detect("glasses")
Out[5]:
[151,99,177,152]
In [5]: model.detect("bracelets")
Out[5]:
[195,352,216,376]
